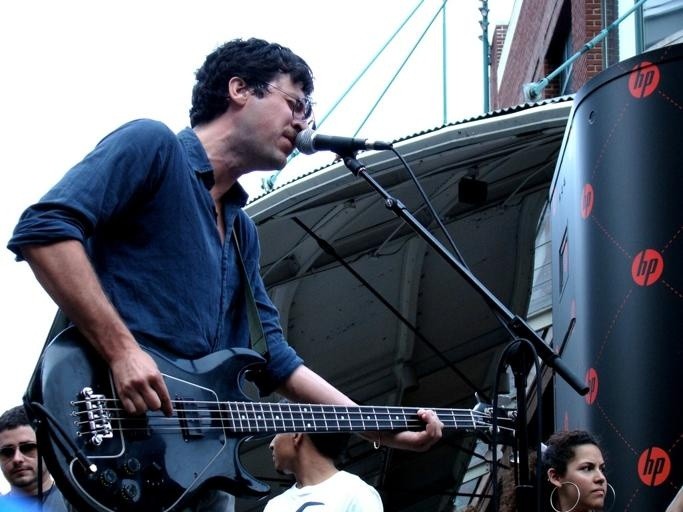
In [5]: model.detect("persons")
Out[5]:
[3,33,443,512]
[0,404,74,512]
[529,430,608,510]
[261,395,384,512]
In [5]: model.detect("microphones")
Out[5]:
[296,128,393,155]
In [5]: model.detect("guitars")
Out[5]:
[24,326,518,512]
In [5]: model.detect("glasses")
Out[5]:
[265,81,316,130]
[0,444,37,458]
